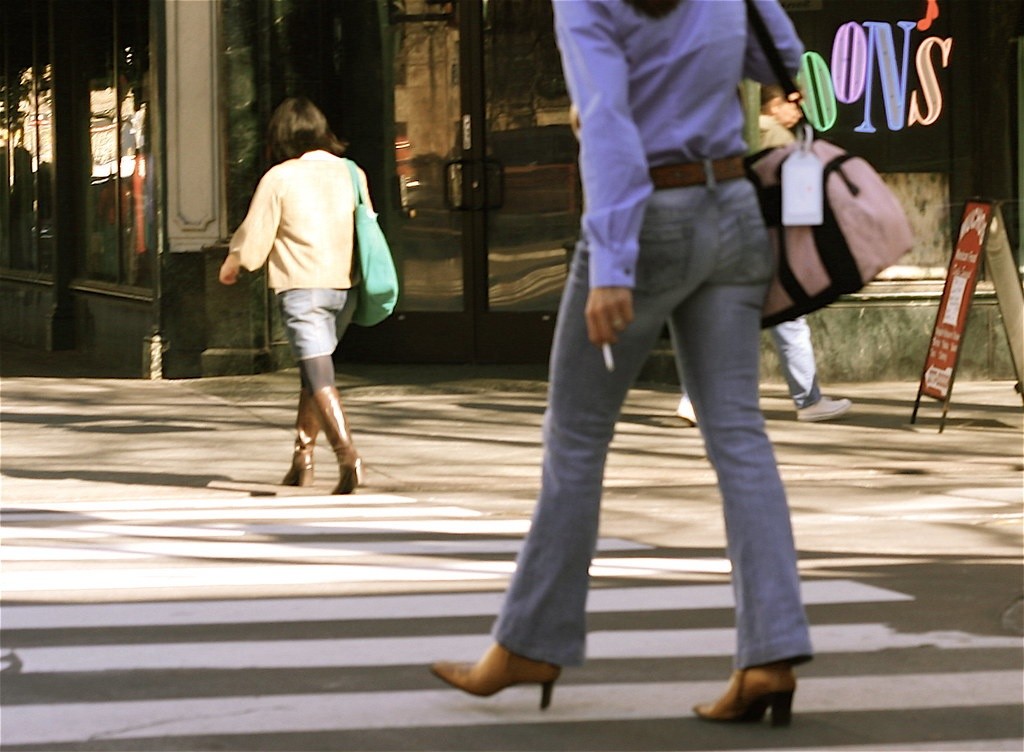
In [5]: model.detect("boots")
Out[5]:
[308,385,362,495]
[282,388,321,488]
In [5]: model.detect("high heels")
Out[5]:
[693,668,796,727]
[427,648,560,710]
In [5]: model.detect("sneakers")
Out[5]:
[795,395,852,421]
[676,399,697,424]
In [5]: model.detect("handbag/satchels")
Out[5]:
[346,159,399,325]
[743,133,909,329]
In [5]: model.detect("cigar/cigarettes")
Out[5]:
[601,342,615,373]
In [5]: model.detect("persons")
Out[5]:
[676,80,852,427]
[219,97,375,494]
[428,0,813,728]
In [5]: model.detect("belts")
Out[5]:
[647,152,746,188]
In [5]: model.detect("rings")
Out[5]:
[611,319,622,327]
